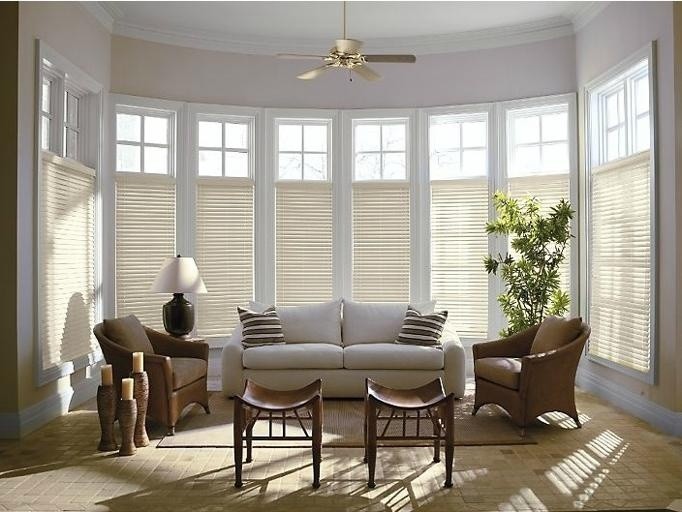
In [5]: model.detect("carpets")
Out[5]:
[158,383,539,450]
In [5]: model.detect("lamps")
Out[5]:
[150,253,209,337]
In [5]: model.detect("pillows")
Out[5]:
[101,312,153,355]
[529,314,583,357]
[395,304,449,344]
[233,304,286,348]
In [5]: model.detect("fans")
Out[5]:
[274,1,416,86]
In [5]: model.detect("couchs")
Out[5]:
[219,295,468,409]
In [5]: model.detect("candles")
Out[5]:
[101,361,112,386]
[131,350,145,373]
[119,378,136,401]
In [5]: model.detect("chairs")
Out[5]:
[91,315,211,433]
[471,317,594,443]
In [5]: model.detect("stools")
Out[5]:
[226,376,329,486]
[360,372,457,488]
